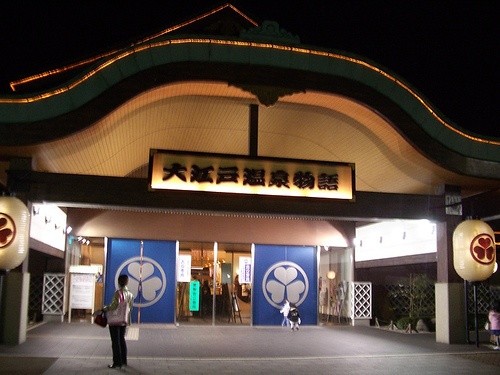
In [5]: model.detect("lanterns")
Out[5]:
[453,216,496,285]
[0,192,30,275]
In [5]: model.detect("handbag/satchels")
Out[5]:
[298,316,301,325]
[108,291,131,327]
[94,312,108,328]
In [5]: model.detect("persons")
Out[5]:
[103,274,133,368]
[280,299,300,331]
[489,305,500,349]
[234,275,239,288]
[193,277,210,315]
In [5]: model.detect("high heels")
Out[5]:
[107,360,127,369]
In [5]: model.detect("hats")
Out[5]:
[289,303,296,308]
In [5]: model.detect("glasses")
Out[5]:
[290,306,292,307]
